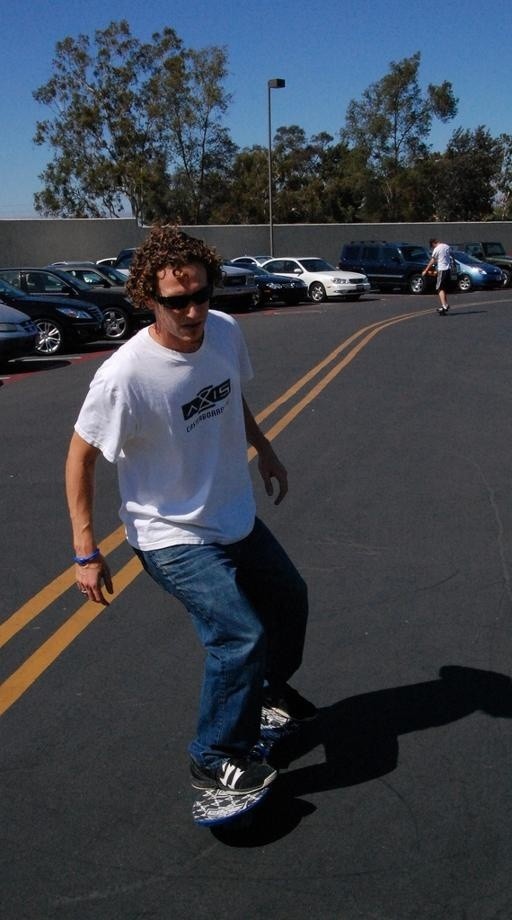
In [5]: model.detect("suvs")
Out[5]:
[448,242,512,288]
[338,240,438,295]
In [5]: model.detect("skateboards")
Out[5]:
[192,702,302,826]
[437,307,449,315]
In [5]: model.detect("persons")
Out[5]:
[63,229,318,796]
[422,238,456,311]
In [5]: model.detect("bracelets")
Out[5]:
[73,550,101,566]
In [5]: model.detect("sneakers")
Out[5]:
[438,305,448,311]
[191,755,278,795]
[263,684,318,722]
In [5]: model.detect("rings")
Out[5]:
[81,590,89,598]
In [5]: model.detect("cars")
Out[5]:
[411,251,505,293]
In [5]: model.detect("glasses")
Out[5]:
[151,283,213,310]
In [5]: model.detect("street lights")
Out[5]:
[267,78,286,258]
